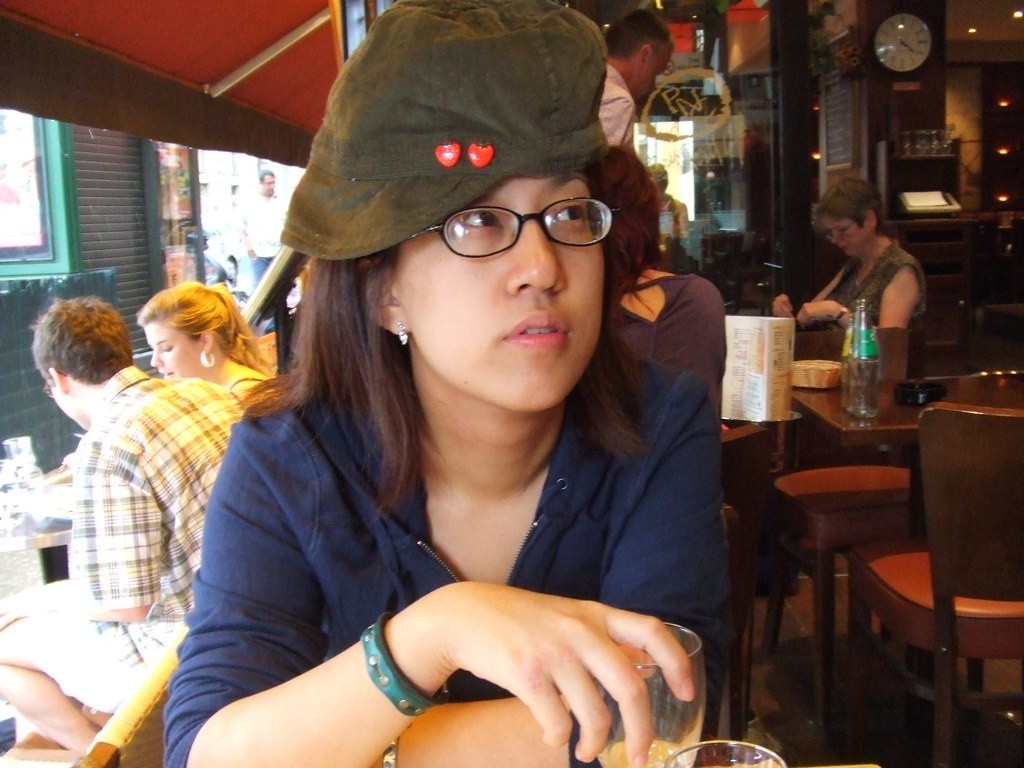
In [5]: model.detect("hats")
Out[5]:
[279,0,607,260]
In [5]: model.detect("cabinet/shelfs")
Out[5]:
[875,138,979,336]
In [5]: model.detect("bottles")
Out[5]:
[841,298,881,419]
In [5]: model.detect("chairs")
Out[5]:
[720,326,1024,768]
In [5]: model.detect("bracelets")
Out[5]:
[383,737,397,768]
[361,612,453,715]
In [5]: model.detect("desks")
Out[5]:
[792,375,1024,735]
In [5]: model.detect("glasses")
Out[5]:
[825,214,869,243]
[42,370,68,399]
[408,197,622,258]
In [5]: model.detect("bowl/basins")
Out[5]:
[893,383,945,406]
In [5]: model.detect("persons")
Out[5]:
[599,9,673,151]
[770,178,925,329]
[0,295,247,750]
[602,145,725,426]
[242,170,287,288]
[135,281,269,396]
[649,164,689,238]
[164,0,731,768]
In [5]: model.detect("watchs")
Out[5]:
[837,307,848,319]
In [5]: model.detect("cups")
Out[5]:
[0,435,44,529]
[665,739,788,768]
[592,622,706,768]
[900,129,952,156]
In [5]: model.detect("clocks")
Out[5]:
[872,12,932,73]
